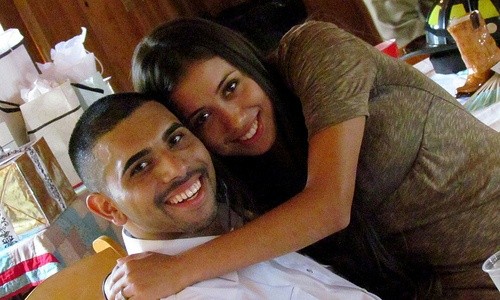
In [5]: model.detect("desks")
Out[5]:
[0,185,129,300]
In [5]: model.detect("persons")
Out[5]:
[99,16,499,300]
[68,93,386,300]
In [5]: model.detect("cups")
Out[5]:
[374,38,398,59]
[447,9,500,82]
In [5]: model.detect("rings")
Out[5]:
[118,289,129,300]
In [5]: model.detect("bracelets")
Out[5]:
[101,272,112,300]
